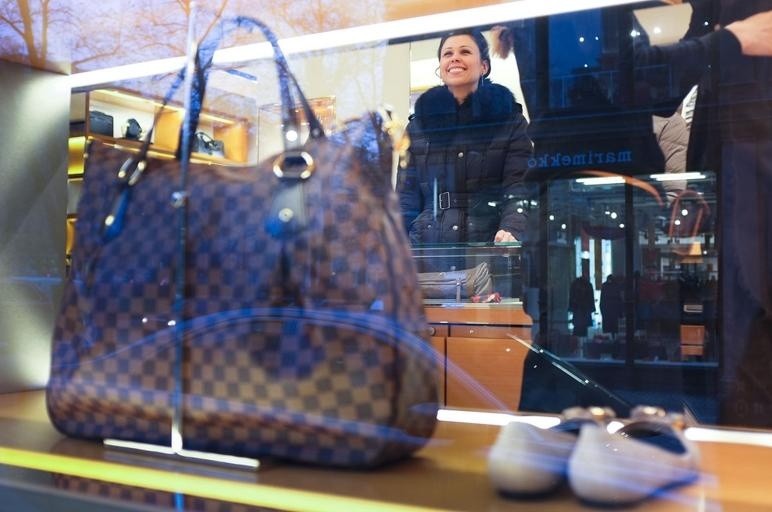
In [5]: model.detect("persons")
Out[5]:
[652,2,771,399]
[569,277,596,338]
[395,29,535,248]
[599,275,624,339]
[491,0,771,172]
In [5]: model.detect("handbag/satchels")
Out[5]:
[638,272,667,301]
[122,118,146,142]
[416,261,492,299]
[71,111,114,137]
[191,131,225,160]
[45,16,440,469]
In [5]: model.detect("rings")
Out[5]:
[506,229,514,239]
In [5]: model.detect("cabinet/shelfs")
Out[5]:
[68,87,249,273]
[395,238,537,413]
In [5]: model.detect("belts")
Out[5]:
[563,169,665,209]
[422,192,489,209]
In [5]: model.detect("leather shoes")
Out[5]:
[488,405,624,496]
[567,403,701,505]
